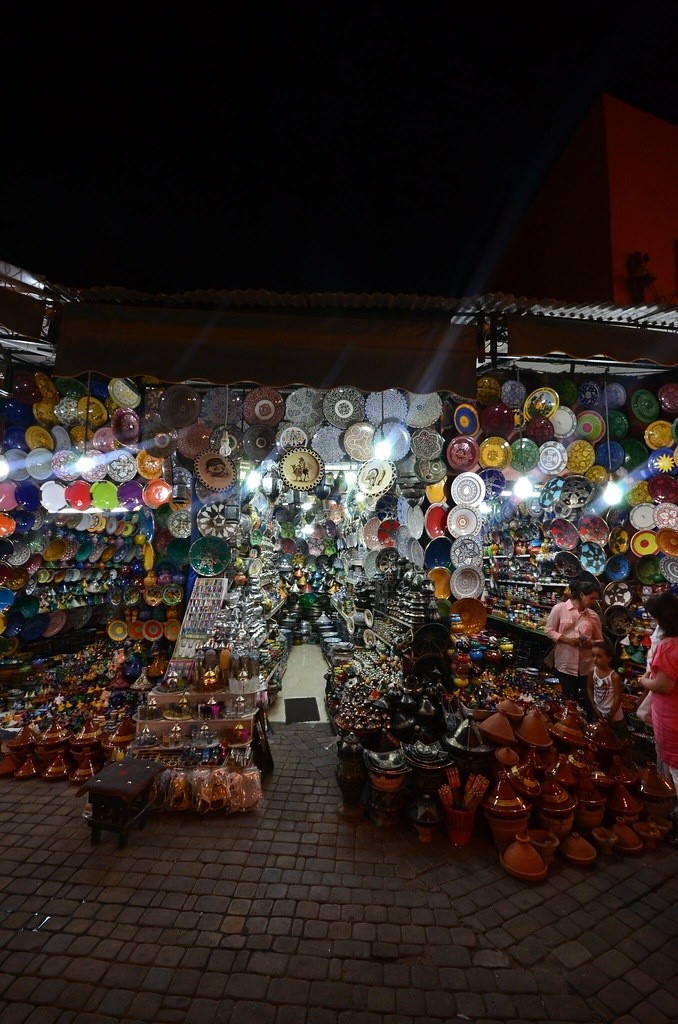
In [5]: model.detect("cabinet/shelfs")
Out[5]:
[131,684,266,766]
[260,577,415,688]
[480,553,571,640]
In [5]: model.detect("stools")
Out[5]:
[77,759,165,847]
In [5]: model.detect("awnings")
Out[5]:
[36,274,678,336]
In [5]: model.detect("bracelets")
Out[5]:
[638,677,641,683]
[608,711,612,716]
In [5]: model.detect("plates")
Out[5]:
[107,620,128,641]
[128,621,145,640]
[74,606,92,631]
[0,659,23,668]
[450,597,487,636]
[164,619,181,641]
[20,613,50,643]
[412,623,452,656]
[42,611,66,637]
[604,606,633,637]
[0,370,678,618]
[3,612,25,637]
[0,636,19,657]
[0,612,7,634]
[142,620,163,641]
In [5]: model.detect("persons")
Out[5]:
[587,641,625,740]
[631,590,678,847]
[544,571,604,721]
[637,596,671,781]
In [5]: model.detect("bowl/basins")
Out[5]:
[592,828,618,853]
[527,830,560,864]
[634,815,672,849]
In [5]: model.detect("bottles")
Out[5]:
[107,646,259,766]
